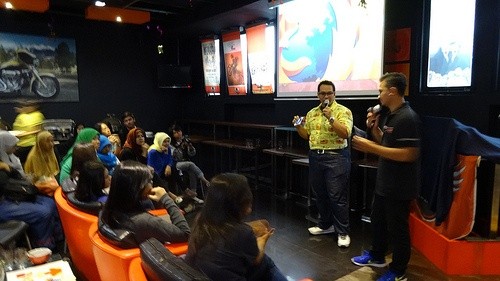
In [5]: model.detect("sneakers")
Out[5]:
[337,232,350,247]
[350,250,386,267]
[308,226,335,235]
[376,271,407,281]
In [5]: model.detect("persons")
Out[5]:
[293,80,354,247]
[351,71,421,281]
[0,97,288,281]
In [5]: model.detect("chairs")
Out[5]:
[0,178,211,281]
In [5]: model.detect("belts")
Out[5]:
[313,149,343,154]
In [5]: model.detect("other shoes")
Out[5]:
[191,196,205,204]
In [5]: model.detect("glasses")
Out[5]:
[319,92,334,96]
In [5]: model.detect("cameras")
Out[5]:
[182,135,191,143]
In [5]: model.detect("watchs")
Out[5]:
[328,117,335,124]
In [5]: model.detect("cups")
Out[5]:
[27,248,51,264]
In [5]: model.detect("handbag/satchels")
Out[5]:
[5,183,36,203]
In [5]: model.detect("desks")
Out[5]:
[182,119,380,228]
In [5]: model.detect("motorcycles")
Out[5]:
[0,49,60,100]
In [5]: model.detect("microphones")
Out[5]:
[323,99,329,116]
[370,104,382,128]
